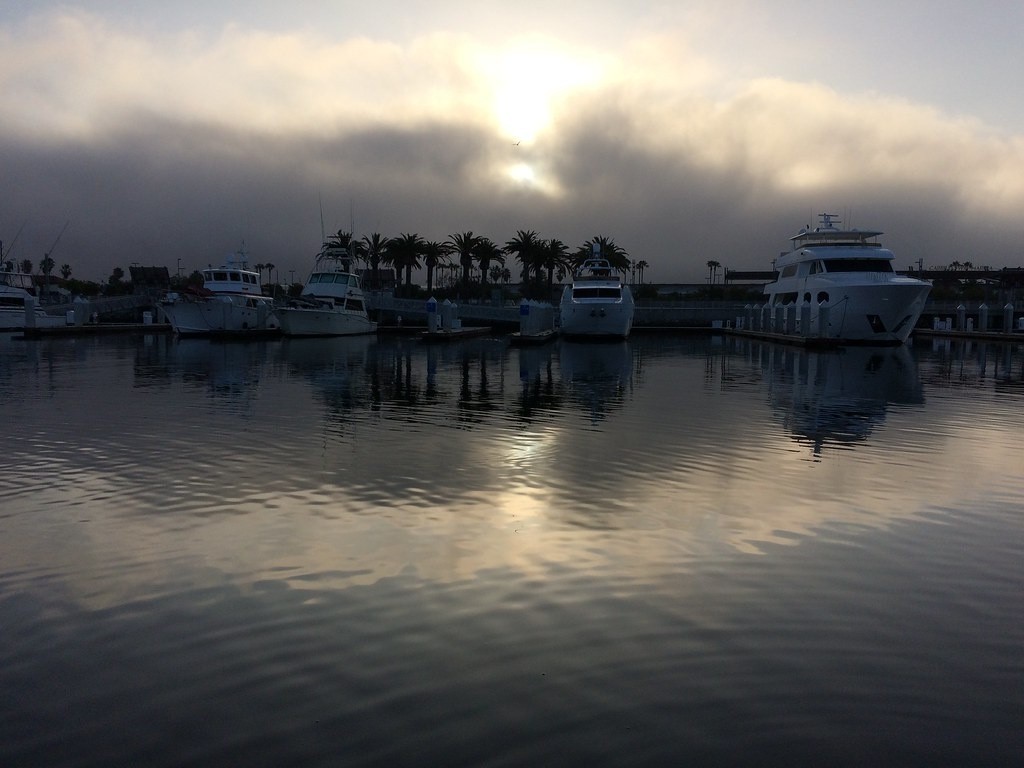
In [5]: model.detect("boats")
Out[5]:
[0,271,67,330]
[155,237,280,335]
[757,212,933,346]
[560,242,635,340]
[271,257,378,336]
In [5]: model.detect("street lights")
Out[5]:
[131,263,139,267]
[288,270,296,285]
[177,259,182,285]
